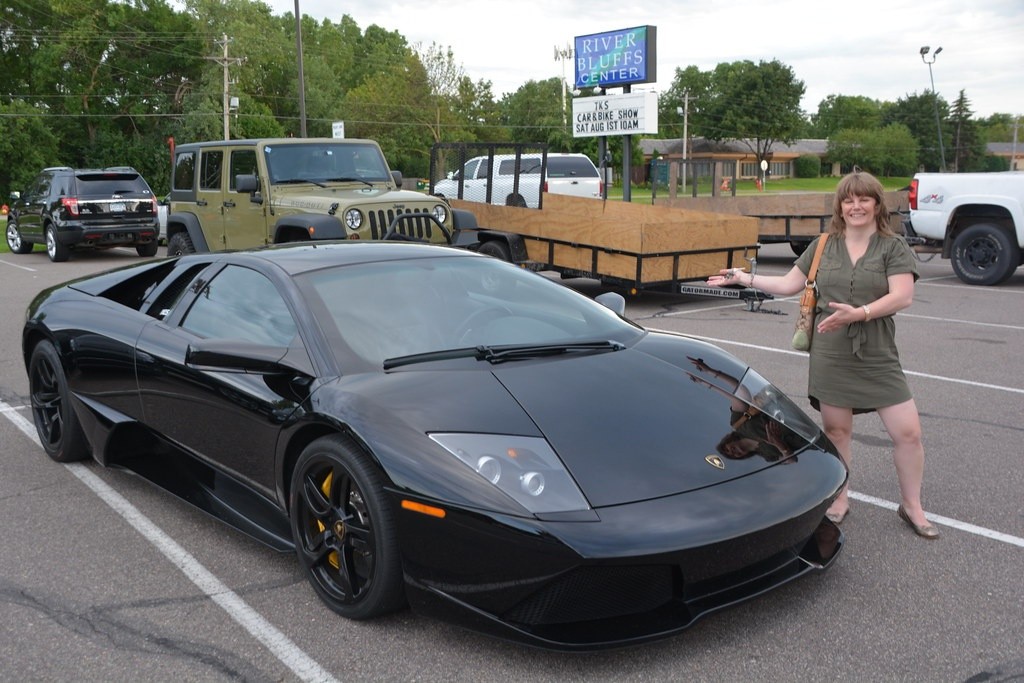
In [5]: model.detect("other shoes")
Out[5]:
[896,503,940,539]
[825,505,849,525]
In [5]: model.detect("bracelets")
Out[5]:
[749,273,755,288]
[862,305,871,322]
[783,448,790,454]
[714,370,721,377]
[708,384,713,390]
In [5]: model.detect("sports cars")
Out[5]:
[20,241,854,652]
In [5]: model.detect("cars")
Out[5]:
[156,192,169,246]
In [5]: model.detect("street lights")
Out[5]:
[919,45,944,172]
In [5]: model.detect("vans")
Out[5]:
[434,151,603,210]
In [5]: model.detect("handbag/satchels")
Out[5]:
[790,231,830,352]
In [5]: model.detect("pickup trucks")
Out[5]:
[901,169,1024,287]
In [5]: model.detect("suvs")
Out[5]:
[165,137,482,259]
[5,166,161,263]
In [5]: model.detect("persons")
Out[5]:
[706,172,941,537]
[686,356,840,465]
